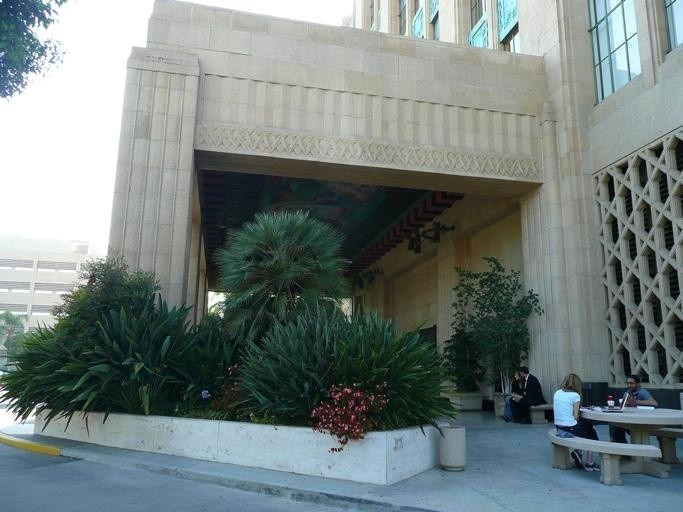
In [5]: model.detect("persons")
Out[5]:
[501,372,524,423]
[552,373,600,472]
[509,366,546,424]
[608,375,658,460]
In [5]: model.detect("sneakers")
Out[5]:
[571,449,583,468]
[585,462,600,472]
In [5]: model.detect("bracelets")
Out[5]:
[637,399,640,406]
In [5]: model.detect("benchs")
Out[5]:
[500,396,549,425]
[549,401,683,486]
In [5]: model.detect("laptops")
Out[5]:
[601,391,629,412]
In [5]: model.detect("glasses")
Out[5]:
[626,382,637,385]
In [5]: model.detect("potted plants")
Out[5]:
[442,255,546,416]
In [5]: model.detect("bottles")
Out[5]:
[606,395,614,408]
